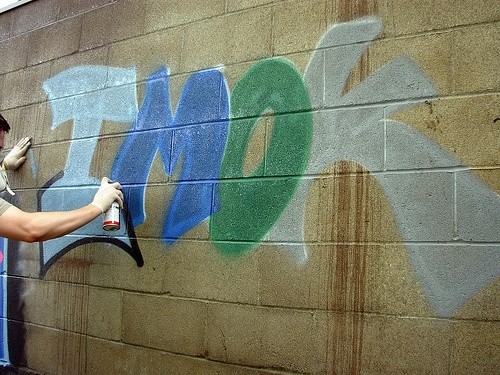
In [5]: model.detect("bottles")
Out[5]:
[102,182,121,232]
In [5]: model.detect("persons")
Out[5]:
[0,115,123,242]
